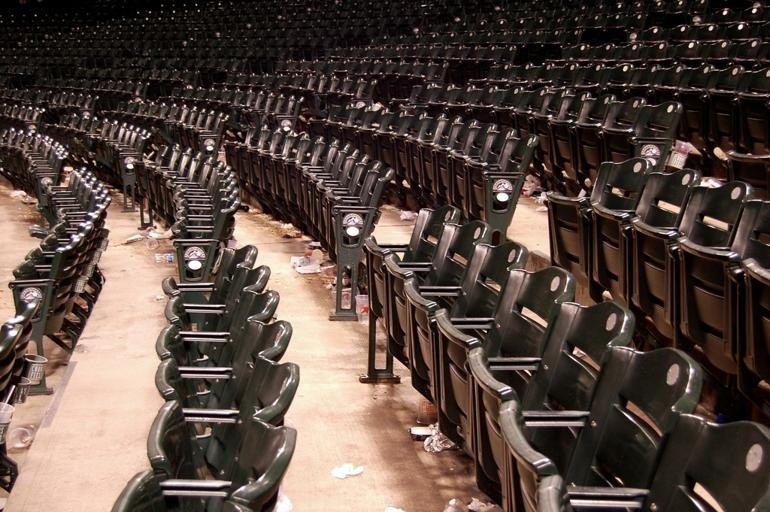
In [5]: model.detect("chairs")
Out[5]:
[0,0,769,512]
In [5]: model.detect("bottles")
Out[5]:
[155,253,177,265]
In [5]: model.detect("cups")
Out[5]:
[443,498,469,512]
[416,397,437,424]
[9,424,37,448]
[354,294,370,325]
[0,402,15,445]
[147,237,159,250]
[676,139,692,156]
